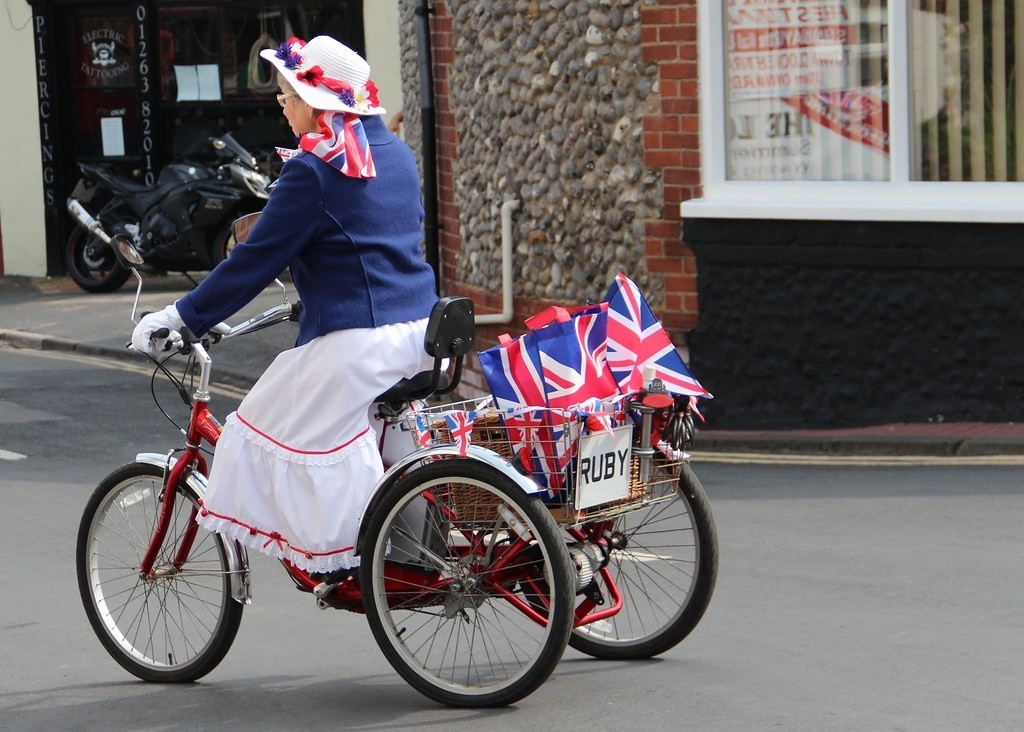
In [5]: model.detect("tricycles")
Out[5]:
[73,207,721,710]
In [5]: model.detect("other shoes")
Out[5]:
[309,566,358,586]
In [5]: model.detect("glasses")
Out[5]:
[276,90,297,108]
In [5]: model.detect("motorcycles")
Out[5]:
[64,131,276,295]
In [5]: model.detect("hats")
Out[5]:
[260,35,387,116]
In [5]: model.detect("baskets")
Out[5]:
[407,398,691,530]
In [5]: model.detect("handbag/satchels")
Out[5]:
[478,300,632,507]
[572,273,713,445]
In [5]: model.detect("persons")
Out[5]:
[130,34,444,580]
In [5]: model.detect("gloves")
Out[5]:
[131,299,188,353]
